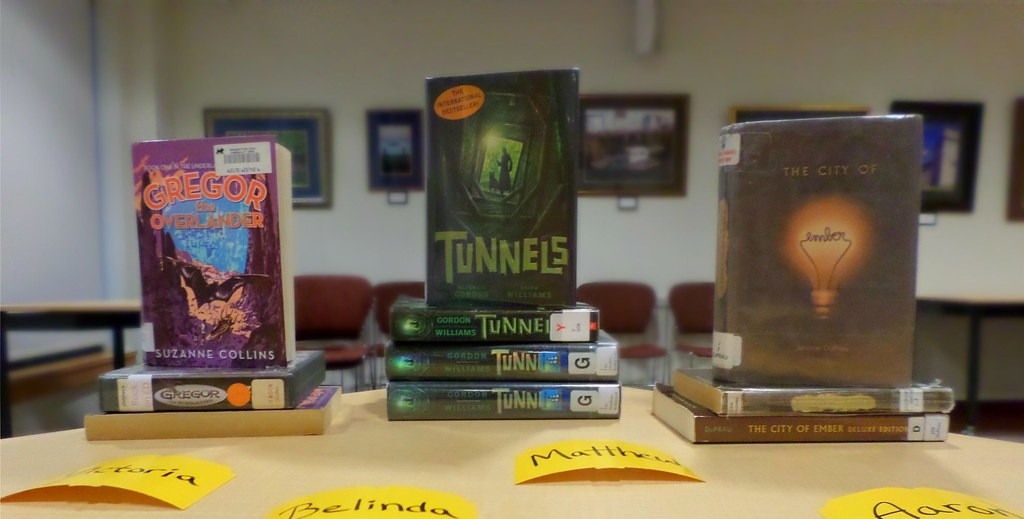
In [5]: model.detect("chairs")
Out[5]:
[667,282,715,368]
[364,282,427,390]
[292,274,377,396]
[576,281,668,386]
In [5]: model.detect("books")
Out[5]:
[384,329,620,382]
[425,68,579,310]
[98,349,326,413]
[713,115,923,387]
[84,386,342,441]
[389,295,600,346]
[131,135,296,370]
[671,368,955,417]
[386,382,622,421]
[651,382,949,444]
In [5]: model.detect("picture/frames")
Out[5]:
[203,109,333,209]
[576,92,691,197]
[366,109,425,192]
[727,104,872,126]
[888,100,984,212]
[1004,97,1023,222]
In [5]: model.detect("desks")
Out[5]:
[2,387,1024,519]
[915,295,1024,438]
[0,301,143,439]
